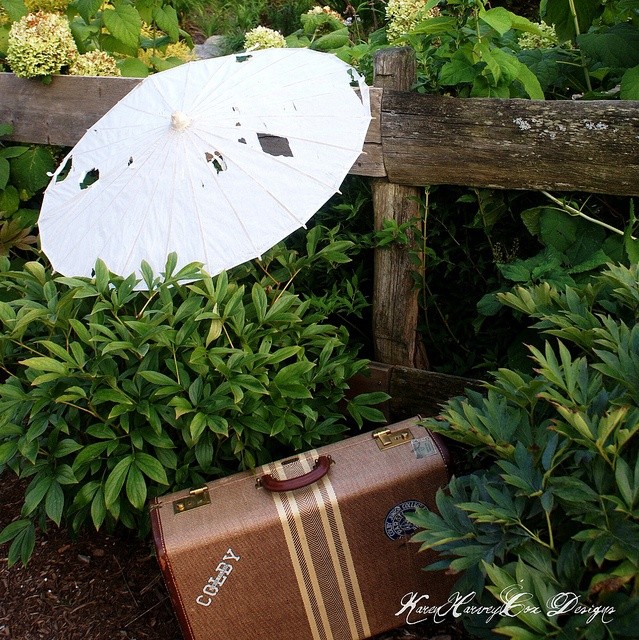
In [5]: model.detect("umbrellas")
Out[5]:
[32,44,377,286]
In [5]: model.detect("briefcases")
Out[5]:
[147,413,466,640]
